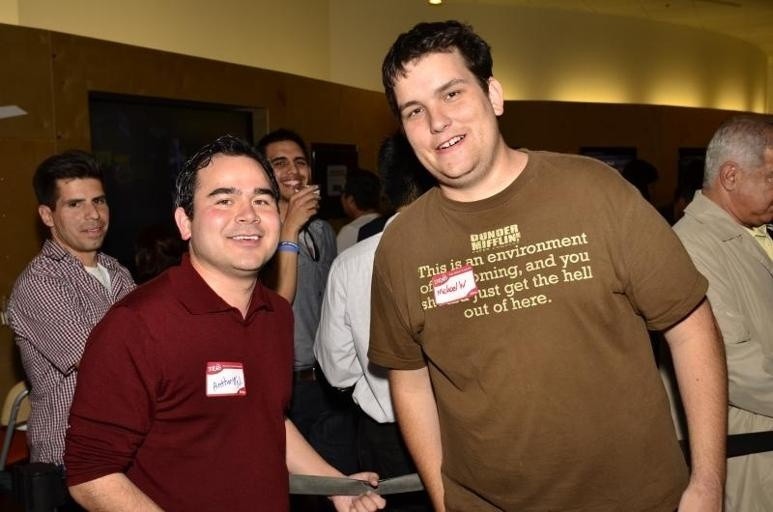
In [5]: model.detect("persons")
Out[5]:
[62,134,386,512]
[254,127,441,482]
[8,151,137,512]
[671,111,771,512]
[363,22,731,512]
[128,218,186,284]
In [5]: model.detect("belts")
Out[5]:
[295,367,320,384]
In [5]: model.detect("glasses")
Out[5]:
[303,225,320,261]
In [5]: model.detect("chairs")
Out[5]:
[2,378,38,494]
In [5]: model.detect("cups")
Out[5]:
[294,184,320,196]
